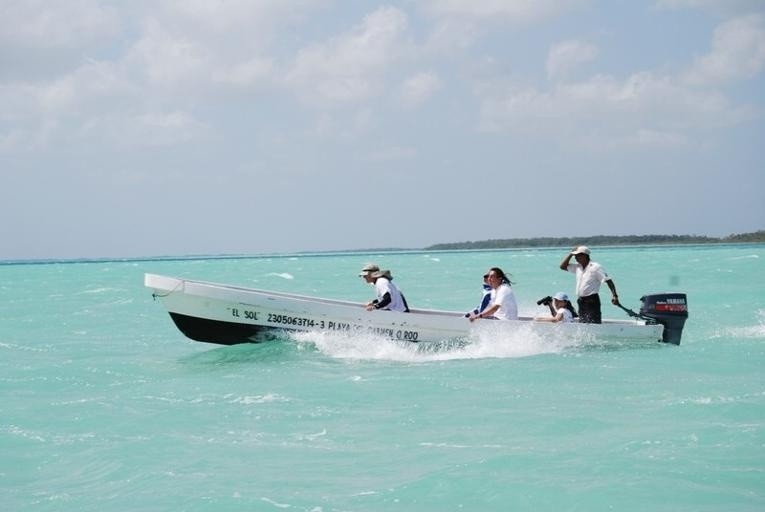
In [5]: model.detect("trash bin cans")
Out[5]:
[640,293,688,345]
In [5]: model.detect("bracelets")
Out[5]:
[613,294,618,298]
[480,314,482,318]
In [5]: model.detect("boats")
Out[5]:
[142,268,693,355]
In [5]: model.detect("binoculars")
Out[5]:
[537,296,553,306]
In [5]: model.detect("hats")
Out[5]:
[553,292,570,302]
[381,269,394,281]
[359,264,383,278]
[571,245,591,256]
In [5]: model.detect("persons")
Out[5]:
[359,264,407,313]
[380,270,409,312]
[534,292,578,324]
[559,245,618,324]
[465,273,495,318]
[469,267,517,322]
